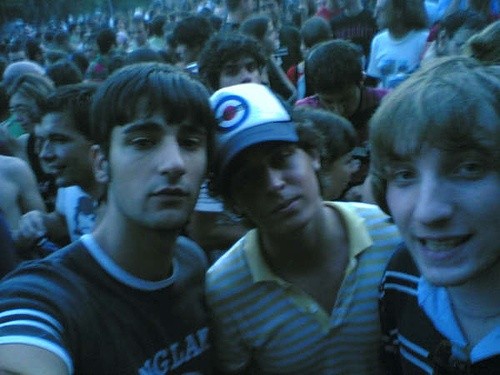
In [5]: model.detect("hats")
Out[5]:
[208,81,306,180]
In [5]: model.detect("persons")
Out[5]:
[200,80,407,375]
[366,54,500,375]
[0,0,500,282]
[0,61,215,375]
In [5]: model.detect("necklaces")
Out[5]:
[446,287,500,320]
